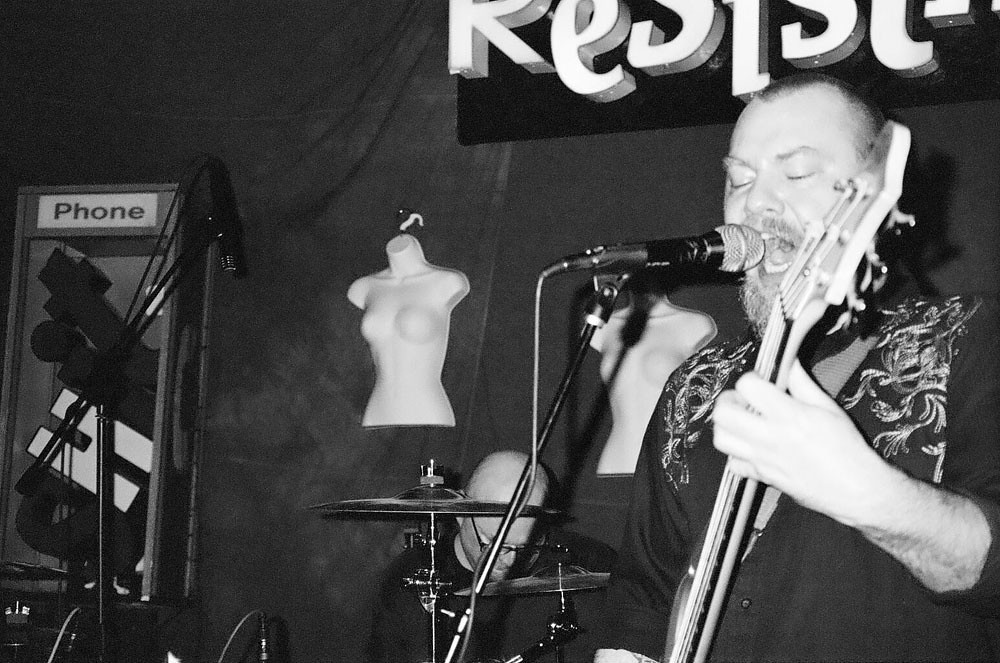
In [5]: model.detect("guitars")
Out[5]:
[659,117,915,663]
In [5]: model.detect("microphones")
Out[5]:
[209,157,243,273]
[539,224,766,278]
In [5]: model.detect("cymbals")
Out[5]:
[454,562,611,597]
[300,485,563,522]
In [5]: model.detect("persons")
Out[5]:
[364,446,622,663]
[345,232,470,429]
[592,69,1000,663]
[588,273,720,477]
